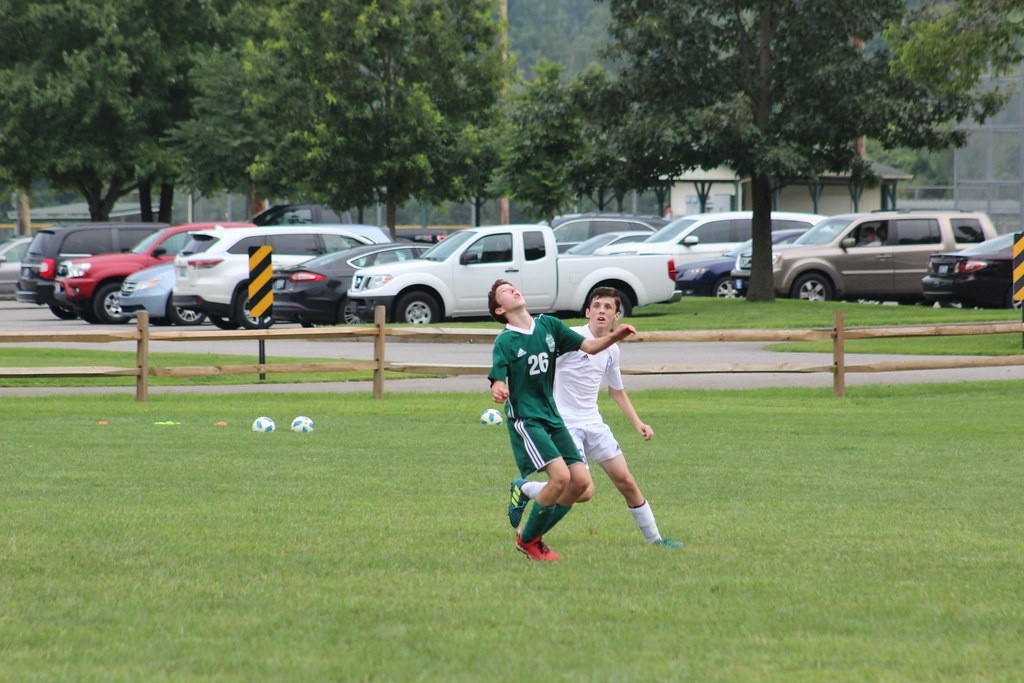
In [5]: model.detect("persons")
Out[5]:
[855,227,882,247]
[487,278,637,560]
[508,287,685,548]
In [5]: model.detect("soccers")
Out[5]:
[291,415,315,433]
[480,408,503,426]
[252,416,276,432]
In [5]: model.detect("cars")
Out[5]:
[921,231,1024,309]
[254,203,684,259]
[119,260,209,326]
[674,227,839,299]
[0,236,34,296]
[271,241,440,328]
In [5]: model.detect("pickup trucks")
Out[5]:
[364,224,677,325]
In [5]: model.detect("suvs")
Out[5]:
[174,221,413,329]
[729,209,998,306]
[15,221,193,320]
[593,211,837,273]
[53,222,275,325]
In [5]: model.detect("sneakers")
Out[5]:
[652,536,684,549]
[507,477,531,528]
[515,531,558,563]
